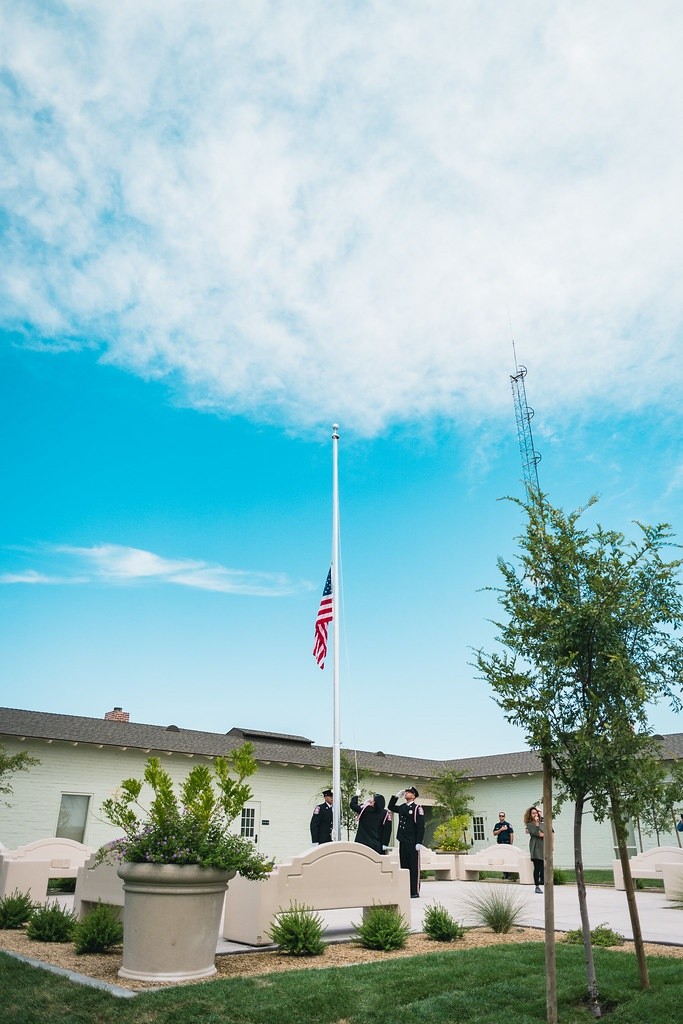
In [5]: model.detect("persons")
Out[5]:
[310,790,334,845]
[349,789,392,855]
[388,786,423,898]
[524,808,556,894]
[493,812,514,879]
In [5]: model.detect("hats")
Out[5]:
[322,788,333,797]
[405,786,419,798]
[374,794,385,809]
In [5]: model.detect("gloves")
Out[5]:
[382,845,388,850]
[312,843,319,847]
[355,788,362,796]
[396,790,405,799]
[415,844,422,851]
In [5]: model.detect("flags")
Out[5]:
[312,566,334,669]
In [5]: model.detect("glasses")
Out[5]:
[499,815,504,817]
[538,810,541,812]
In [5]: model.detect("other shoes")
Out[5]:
[411,895,420,898]
[535,887,543,893]
